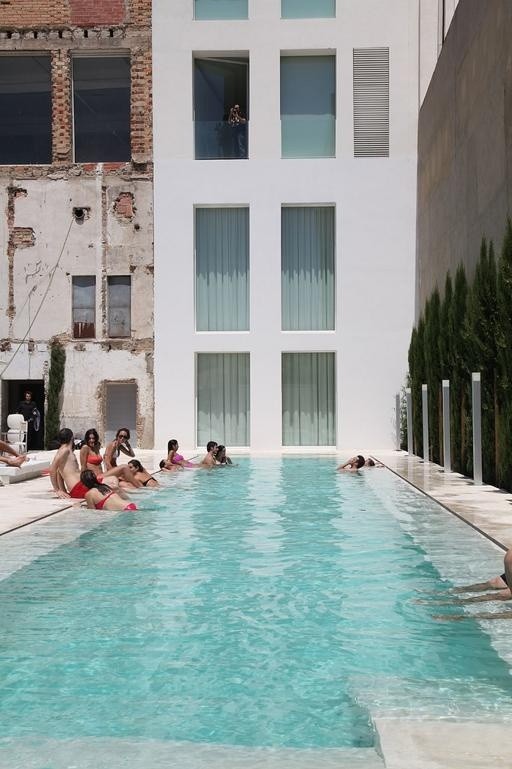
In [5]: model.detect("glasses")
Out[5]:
[120,435,128,439]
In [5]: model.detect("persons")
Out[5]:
[413,549,512,622]
[228,103,246,159]
[0,440,30,467]
[365,459,375,466]
[80,429,129,500]
[80,469,137,511]
[337,455,365,472]
[128,460,159,487]
[160,439,239,472]
[47,428,120,499]
[104,428,136,488]
[17,391,37,451]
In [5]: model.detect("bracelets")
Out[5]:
[127,444,130,448]
